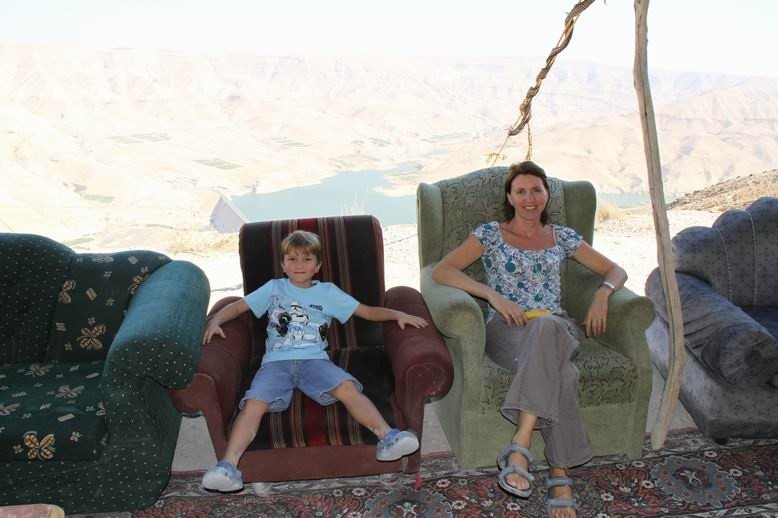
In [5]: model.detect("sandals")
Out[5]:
[376,428,419,461]
[202,460,243,491]
[496,444,534,497]
[546,474,580,518]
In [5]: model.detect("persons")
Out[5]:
[432,161,628,518]
[202,230,428,491]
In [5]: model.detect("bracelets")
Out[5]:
[599,281,615,294]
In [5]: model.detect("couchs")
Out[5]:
[416,166,657,471]
[0,231,211,515]
[644,196,778,446]
[168,214,454,483]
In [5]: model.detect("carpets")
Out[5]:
[66,427,778,518]
